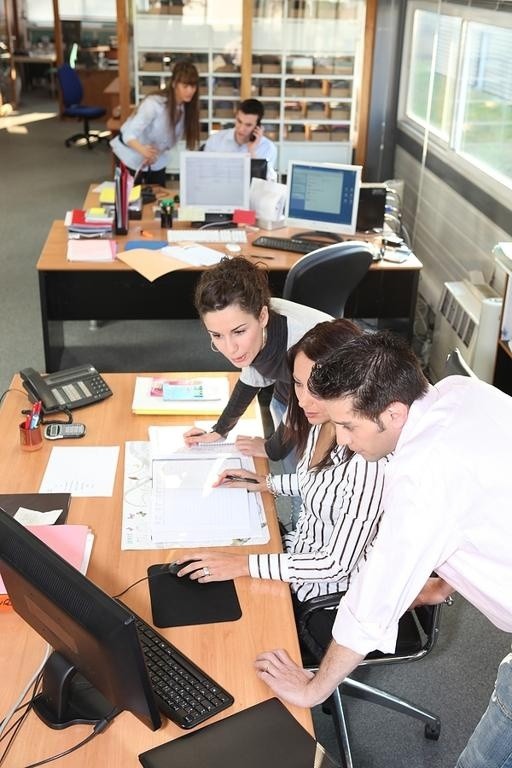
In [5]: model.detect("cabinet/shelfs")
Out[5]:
[135,49,359,143]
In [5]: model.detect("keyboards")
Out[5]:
[114,588,238,727]
[167,229,247,244]
[251,235,323,258]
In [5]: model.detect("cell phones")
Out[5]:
[248,123,264,141]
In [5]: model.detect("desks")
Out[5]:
[36,219,424,372]
[0,369,318,768]
[1,50,58,98]
[82,180,392,233]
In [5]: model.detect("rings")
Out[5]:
[202,566,210,575]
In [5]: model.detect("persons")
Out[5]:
[204,96,278,184]
[176,317,432,709]
[253,331,511,767]
[185,255,341,531]
[108,61,204,188]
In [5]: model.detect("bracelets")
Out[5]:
[434,584,456,607]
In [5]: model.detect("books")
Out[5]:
[131,370,230,415]
[64,161,231,282]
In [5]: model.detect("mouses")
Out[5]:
[225,244,243,253]
[161,556,203,580]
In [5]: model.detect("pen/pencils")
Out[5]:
[252,255,274,260]
[225,475,261,484]
[190,432,207,438]
[25,401,42,429]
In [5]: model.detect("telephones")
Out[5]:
[251,119,261,142]
[19,363,114,416]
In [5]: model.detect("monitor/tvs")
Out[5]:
[0,509,164,739]
[180,150,251,226]
[280,158,364,233]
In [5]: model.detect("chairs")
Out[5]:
[296,572,451,767]
[58,43,109,150]
